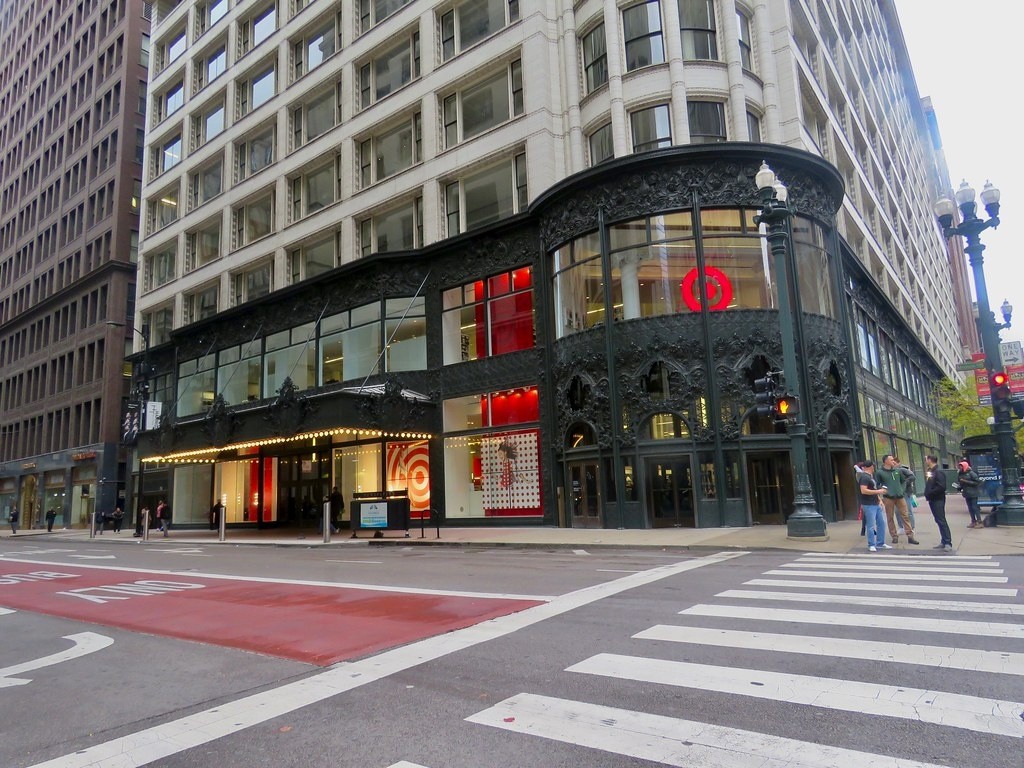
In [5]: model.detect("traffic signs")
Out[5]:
[999,340,1024,367]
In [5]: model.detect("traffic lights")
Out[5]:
[754,376,776,416]
[774,394,799,417]
[1011,396,1024,419]
[990,372,1012,422]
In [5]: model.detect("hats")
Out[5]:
[863,460,873,467]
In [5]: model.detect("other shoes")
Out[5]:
[892,539,898,543]
[944,544,952,551]
[876,543,892,549]
[933,543,944,548]
[897,528,904,534]
[868,546,876,551]
[874,529,876,535]
[966,522,975,527]
[908,538,919,544]
[974,522,984,528]
[861,530,865,535]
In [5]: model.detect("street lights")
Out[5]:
[753,159,827,536]
[932,177,1024,526]
[106,319,151,538]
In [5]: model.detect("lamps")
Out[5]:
[473,387,530,398]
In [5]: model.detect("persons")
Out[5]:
[859,461,892,552]
[10,509,18,533]
[212,499,224,534]
[133,506,142,537]
[318,496,339,535]
[856,462,878,536]
[161,503,172,537]
[876,455,919,544]
[924,454,952,551]
[141,507,151,536]
[45,507,56,532]
[112,508,123,534]
[956,461,983,529]
[893,457,916,535]
[157,500,164,531]
[94,509,106,535]
[329,486,344,532]
